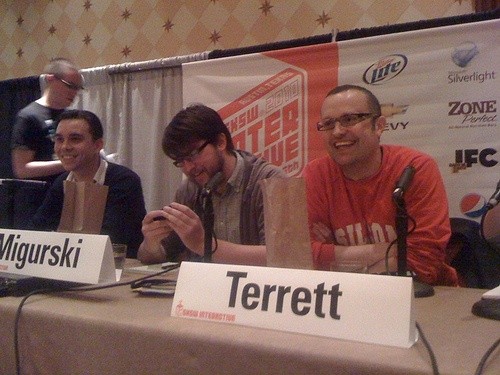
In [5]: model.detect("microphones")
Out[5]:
[201,172,225,198]
[392,166,415,199]
[0,178,51,192]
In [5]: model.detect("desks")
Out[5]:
[0,258,500,375]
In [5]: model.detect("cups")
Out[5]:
[329,260,369,274]
[112,244,127,282]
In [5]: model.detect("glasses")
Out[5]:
[317,112,382,131]
[49,73,84,94]
[173,139,211,168]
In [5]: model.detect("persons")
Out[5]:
[477,181,500,289]
[11,58,83,230]
[137,105,285,266]
[304,85,460,287]
[25,109,146,259]
